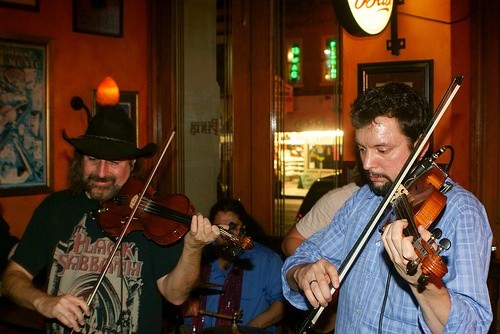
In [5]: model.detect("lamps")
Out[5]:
[71,76,121,124]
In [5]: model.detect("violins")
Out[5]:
[94,175,257,251]
[376,163,452,286]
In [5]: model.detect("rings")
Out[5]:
[309,280,317,285]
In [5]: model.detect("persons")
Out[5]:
[282,176,367,258]
[170,198,285,334]
[281,82,494,334]
[0,107,230,334]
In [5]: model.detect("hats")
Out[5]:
[61,107,159,161]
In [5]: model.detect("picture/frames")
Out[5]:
[357,59,434,157]
[72,0,125,38]
[0,33,54,197]
[92,88,140,150]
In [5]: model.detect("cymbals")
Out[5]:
[193,282,225,288]
[195,324,273,334]
